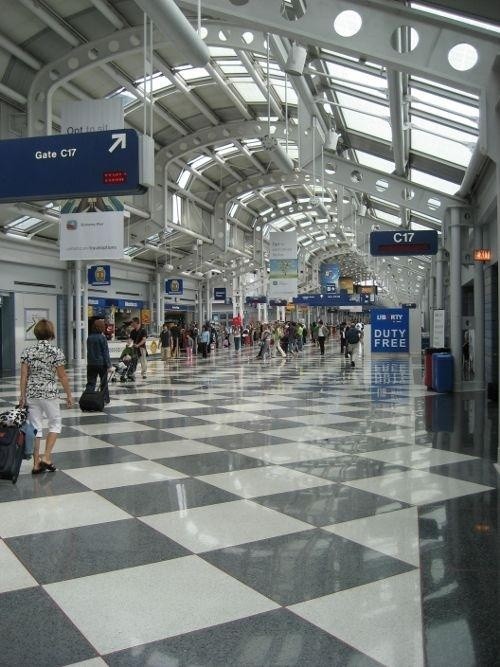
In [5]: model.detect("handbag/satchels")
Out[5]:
[0,406,27,427]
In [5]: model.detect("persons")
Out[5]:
[87,320,112,403]
[158,314,364,369]
[129,317,148,379]
[118,356,129,370]
[20,319,74,474]
[102,320,135,340]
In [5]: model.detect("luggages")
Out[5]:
[0,403,28,484]
[80,370,114,410]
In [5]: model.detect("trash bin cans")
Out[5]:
[425,346,453,393]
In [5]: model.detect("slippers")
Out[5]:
[40,461,56,471]
[32,468,42,474]
